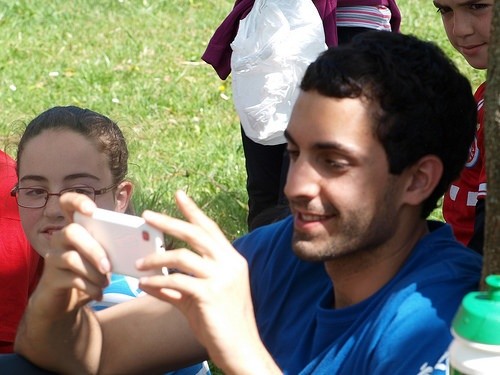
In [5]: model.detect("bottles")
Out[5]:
[447,275,500,375]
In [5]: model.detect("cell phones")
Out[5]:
[71,206,170,280]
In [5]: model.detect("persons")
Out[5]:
[13,30,483,375]
[0,106,212,375]
[201,0,500,256]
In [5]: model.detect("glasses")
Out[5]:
[10,180,123,209]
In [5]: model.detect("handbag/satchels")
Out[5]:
[230,0,330,146]
